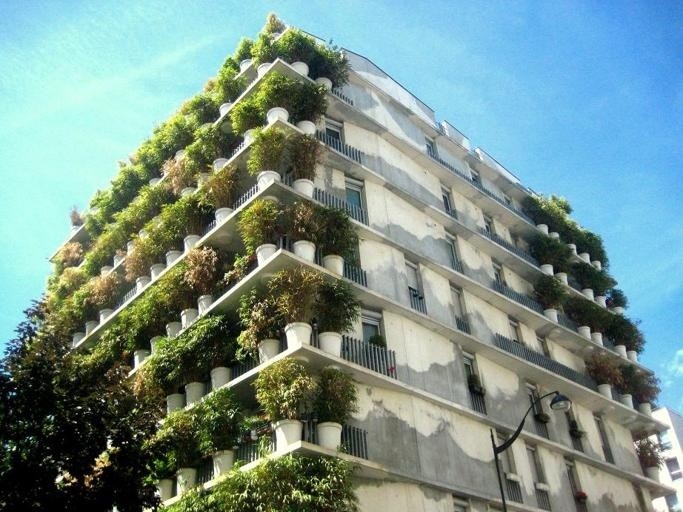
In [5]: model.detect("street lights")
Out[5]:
[489,389,574,512]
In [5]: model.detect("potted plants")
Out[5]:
[311,36,353,93]
[308,365,363,455]
[632,430,662,484]
[310,278,363,361]
[524,190,662,416]
[317,203,361,276]
[39,13,326,503]
[575,492,586,504]
